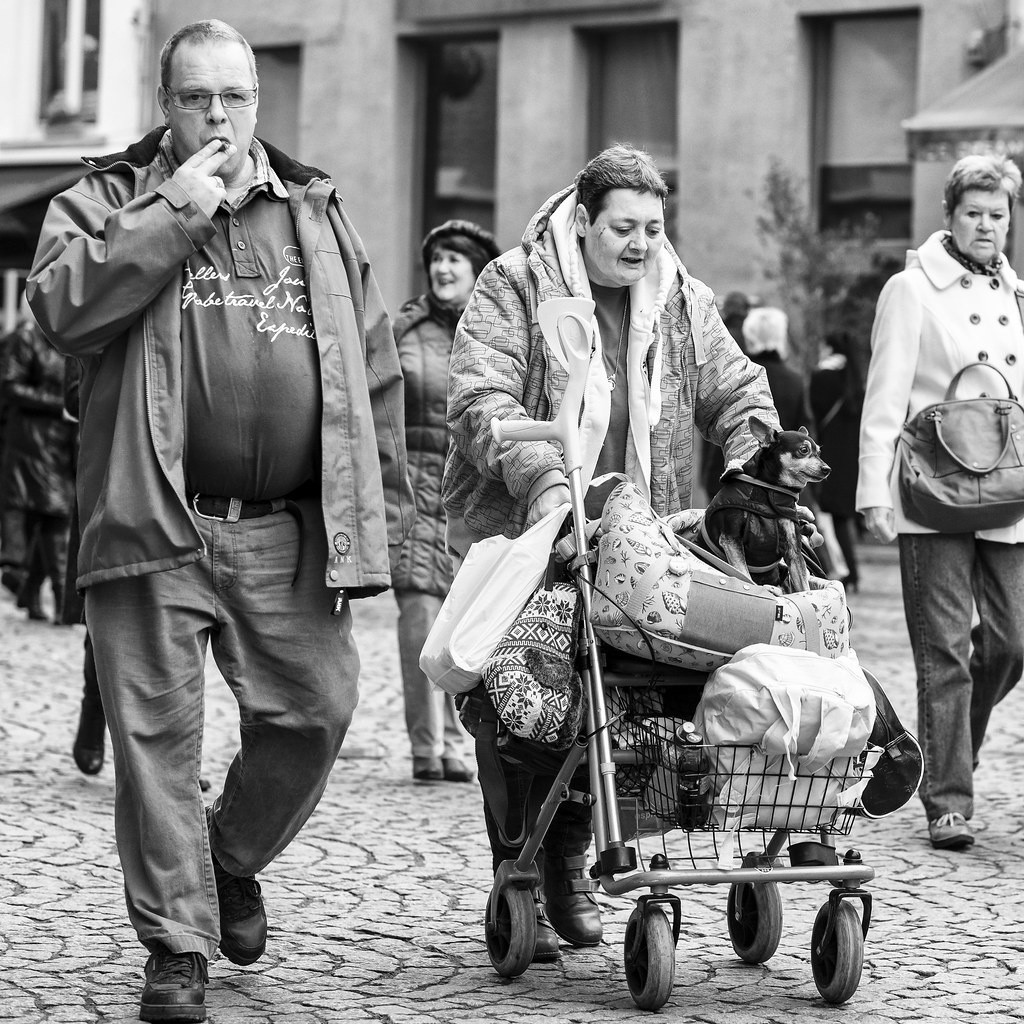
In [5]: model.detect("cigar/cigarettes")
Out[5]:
[218,141,230,152]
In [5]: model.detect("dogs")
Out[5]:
[682,415,832,586]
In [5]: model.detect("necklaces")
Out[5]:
[608,294,629,390]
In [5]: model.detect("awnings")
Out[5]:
[900,47,1024,160]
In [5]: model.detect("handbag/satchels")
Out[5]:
[592,481,848,669]
[417,501,573,697]
[456,683,518,749]
[854,669,925,819]
[890,362,1024,533]
[482,530,584,749]
[690,642,877,831]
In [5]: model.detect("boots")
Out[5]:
[17,574,46,620]
[53,584,65,625]
[74,634,105,774]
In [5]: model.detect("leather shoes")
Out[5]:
[547,854,604,945]
[532,889,560,959]
[212,855,268,966]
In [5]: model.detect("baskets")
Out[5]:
[606,686,884,837]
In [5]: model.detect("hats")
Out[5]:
[724,292,748,316]
[421,220,503,287]
[742,308,788,360]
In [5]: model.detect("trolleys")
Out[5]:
[481,298,877,1012]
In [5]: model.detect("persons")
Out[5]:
[720,289,866,595]
[439,146,784,962]
[0,314,211,793]
[855,154,1024,843]
[387,220,501,783]
[23,18,421,1023]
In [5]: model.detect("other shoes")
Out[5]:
[1,572,23,593]
[412,757,475,786]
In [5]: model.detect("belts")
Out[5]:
[186,486,323,590]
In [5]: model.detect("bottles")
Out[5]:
[645,721,709,833]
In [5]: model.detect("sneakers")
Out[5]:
[930,814,976,847]
[139,943,209,1024]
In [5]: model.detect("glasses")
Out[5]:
[166,84,257,110]
